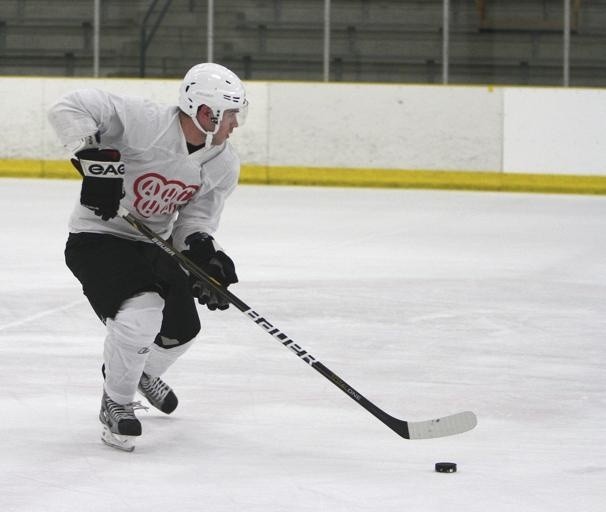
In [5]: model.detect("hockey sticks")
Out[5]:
[118,206,478,440]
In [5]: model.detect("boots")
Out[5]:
[99,363,142,436]
[138,371,178,414]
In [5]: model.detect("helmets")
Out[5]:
[178,62,249,136]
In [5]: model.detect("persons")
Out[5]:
[47,61,251,436]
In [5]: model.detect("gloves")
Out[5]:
[180,231,238,310]
[71,147,126,221]
